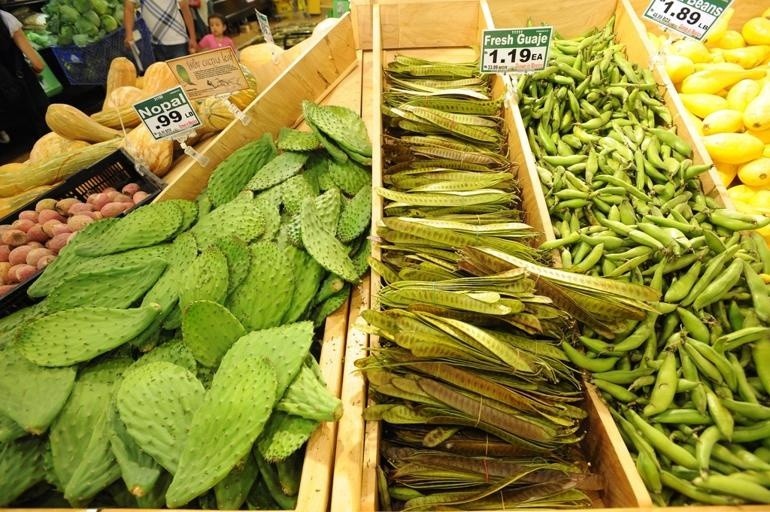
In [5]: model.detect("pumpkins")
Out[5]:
[0,56,257,223]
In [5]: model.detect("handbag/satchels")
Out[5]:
[188,0,201,8]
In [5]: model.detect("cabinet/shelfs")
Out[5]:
[0,0,770,509]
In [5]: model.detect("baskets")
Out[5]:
[0,147,169,319]
[49,17,156,86]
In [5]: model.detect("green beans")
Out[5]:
[350,14,770,512]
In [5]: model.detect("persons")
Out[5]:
[0,11,51,159]
[189,13,235,53]
[122,0,197,72]
